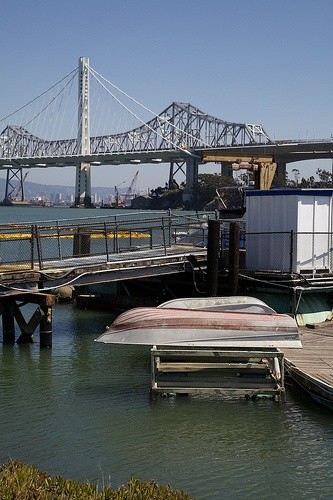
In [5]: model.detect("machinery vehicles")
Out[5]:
[201,154,277,221]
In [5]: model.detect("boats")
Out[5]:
[172,215,208,237]
[93,306,304,350]
[157,295,278,315]
